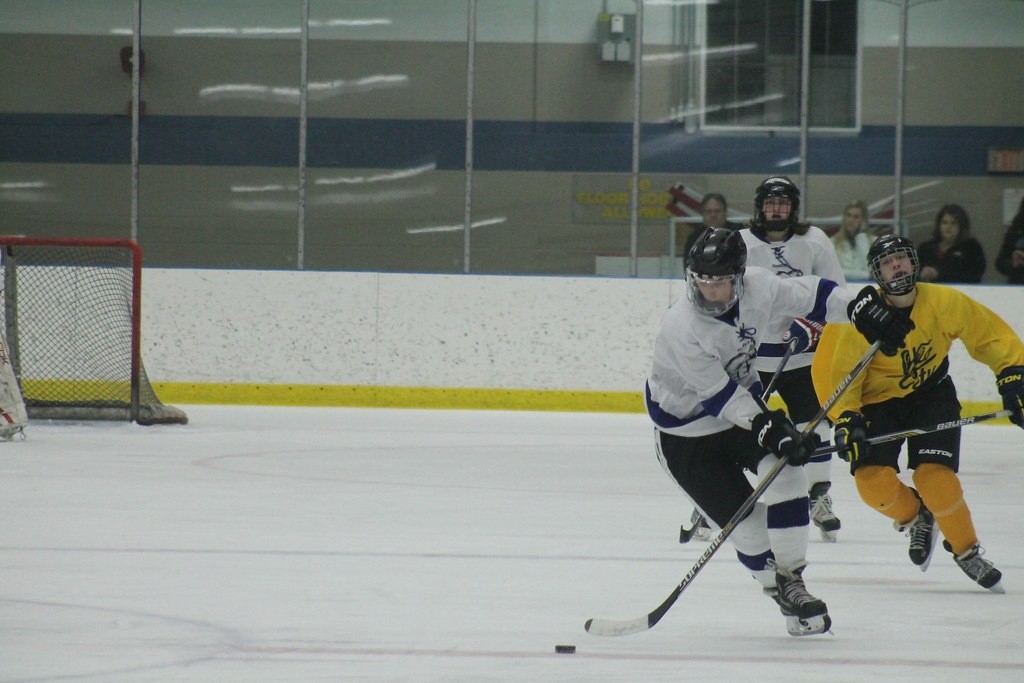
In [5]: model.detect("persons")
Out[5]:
[645,174,1024,639]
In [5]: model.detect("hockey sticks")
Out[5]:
[583,339,882,640]
[671,334,803,547]
[808,409,1018,462]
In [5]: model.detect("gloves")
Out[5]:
[783,318,827,355]
[752,409,821,467]
[996,365,1024,429]
[834,410,871,462]
[847,287,916,356]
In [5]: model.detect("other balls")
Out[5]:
[554,644,578,653]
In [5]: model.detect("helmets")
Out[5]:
[686,226,748,317]
[867,233,920,295]
[755,177,800,230]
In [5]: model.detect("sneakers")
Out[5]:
[808,482,839,543]
[691,507,712,541]
[763,558,831,637]
[894,487,940,572]
[943,539,1006,595]
